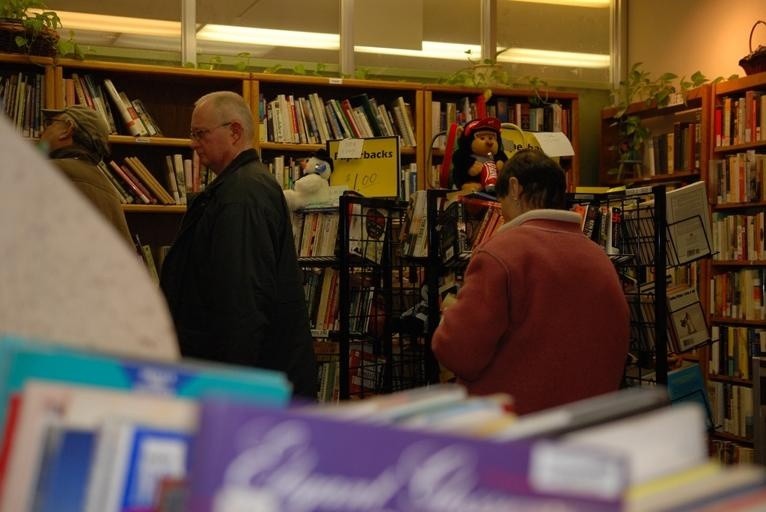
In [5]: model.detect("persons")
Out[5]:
[429,149,630,414]
[158,92,319,403]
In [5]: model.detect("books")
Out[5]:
[573,90,765,355]
[257,92,417,336]
[1,70,219,285]
[432,97,572,309]
[2,336,765,511]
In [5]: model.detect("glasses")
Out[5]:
[185,121,232,141]
[43,118,65,130]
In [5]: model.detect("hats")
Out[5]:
[41,104,109,158]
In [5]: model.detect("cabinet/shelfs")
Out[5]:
[253,72,764,471]
[0,49,252,293]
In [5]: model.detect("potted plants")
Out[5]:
[437,35,555,108]
[606,58,740,182]
[0,1,90,64]
